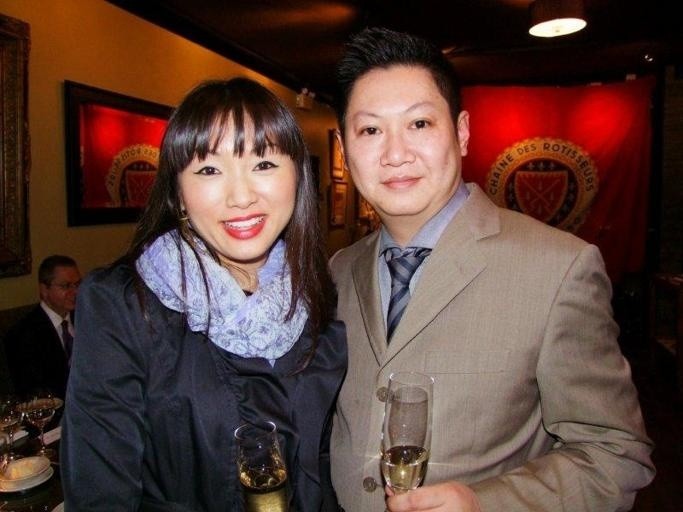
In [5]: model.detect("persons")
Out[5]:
[305,21,658,511]
[45,75,346,511]
[0,254,82,411]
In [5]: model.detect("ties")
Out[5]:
[61,320,73,359]
[384,245,430,344]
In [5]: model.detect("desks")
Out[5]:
[0,399,67,511]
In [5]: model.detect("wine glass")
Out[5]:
[0,398,24,463]
[25,392,57,459]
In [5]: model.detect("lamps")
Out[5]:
[528,0,591,43]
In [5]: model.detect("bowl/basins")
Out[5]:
[0,456,50,483]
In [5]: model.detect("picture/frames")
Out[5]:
[326,127,353,232]
[60,77,179,227]
[0,10,34,281]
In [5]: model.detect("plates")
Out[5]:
[0,467,55,494]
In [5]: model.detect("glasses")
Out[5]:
[49,280,82,289]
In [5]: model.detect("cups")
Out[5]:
[234,420,288,512]
[380,369,434,492]
